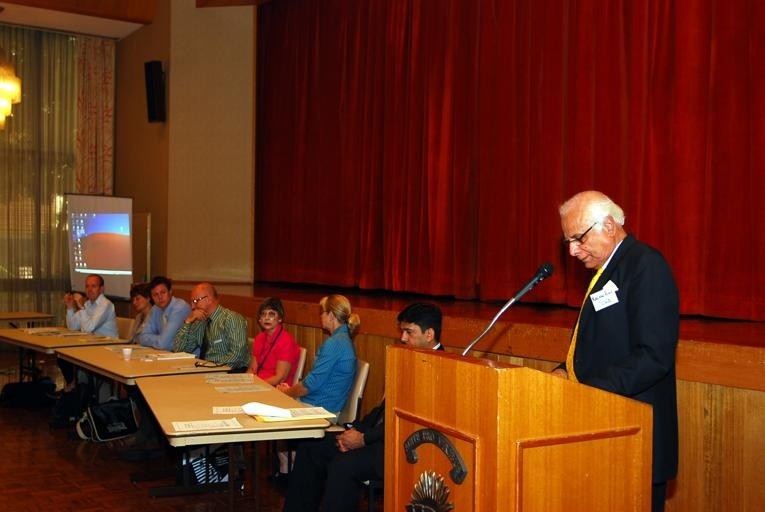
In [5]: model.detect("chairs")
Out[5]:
[294,347,307,382]
[116,315,137,340]
[288,359,370,487]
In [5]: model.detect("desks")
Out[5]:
[1,312,332,512]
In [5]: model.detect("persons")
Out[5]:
[47,274,119,401]
[139,277,200,357]
[266,295,360,489]
[246,296,302,394]
[283,303,445,512]
[550,191,679,512]
[172,282,250,373]
[128,282,155,343]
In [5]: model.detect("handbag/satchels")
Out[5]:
[49,382,95,428]
[176,446,244,493]
[86,396,140,443]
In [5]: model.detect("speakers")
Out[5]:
[144,61,166,120]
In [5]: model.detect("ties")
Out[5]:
[566,268,604,382]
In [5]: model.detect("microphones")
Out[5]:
[462,262,555,355]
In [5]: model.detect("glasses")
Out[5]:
[564,221,598,242]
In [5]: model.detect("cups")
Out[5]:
[122,349,132,360]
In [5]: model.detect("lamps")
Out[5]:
[1,5,22,132]
